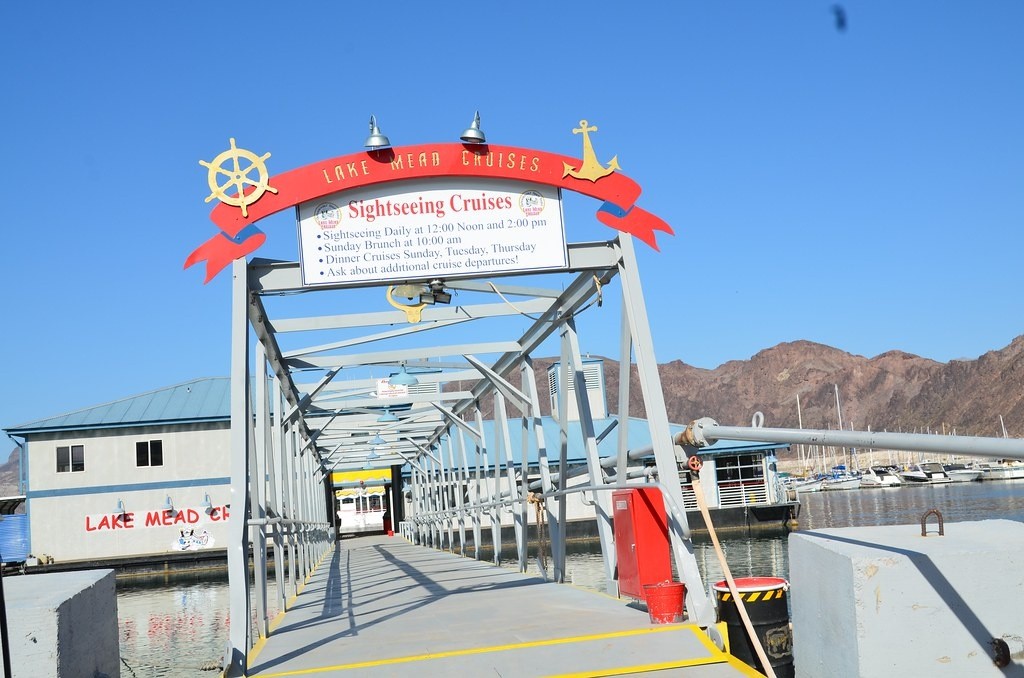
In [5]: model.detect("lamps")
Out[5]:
[460,109,486,144]
[377,409,400,422]
[200,492,212,507]
[420,275,452,305]
[767,450,779,463]
[161,494,173,510]
[364,114,391,150]
[388,363,419,386]
[362,462,374,471]
[367,449,381,459]
[369,432,386,445]
[392,278,427,301]
[112,497,125,514]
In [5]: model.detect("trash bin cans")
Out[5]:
[383,510,391,534]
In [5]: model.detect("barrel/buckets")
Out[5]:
[642,582,686,622]
[387,531,394,536]
[712,577,795,678]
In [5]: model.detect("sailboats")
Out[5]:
[784,383,1024,489]
